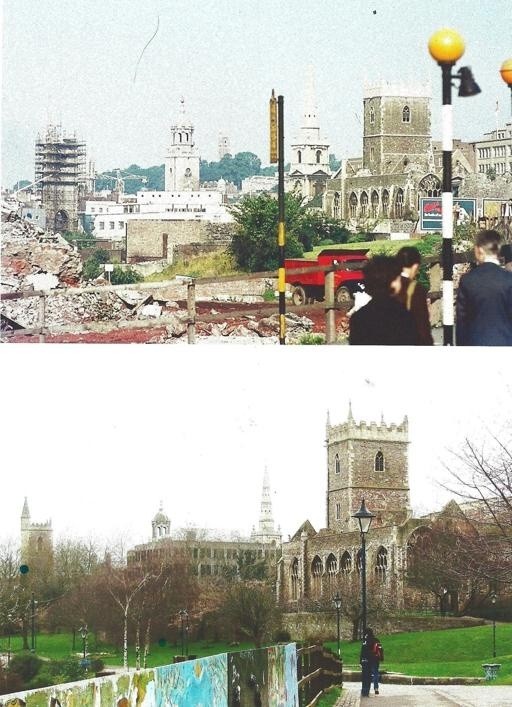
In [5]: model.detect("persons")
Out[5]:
[348,253,428,345]
[454,229,512,346]
[395,246,434,345]
[359,628,381,694]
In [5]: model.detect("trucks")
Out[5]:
[285,249,369,306]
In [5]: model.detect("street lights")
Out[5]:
[431,31,463,341]
[351,497,376,643]
[499,61,511,115]
[488,592,498,656]
[333,593,342,655]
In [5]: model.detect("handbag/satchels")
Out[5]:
[374,642,382,656]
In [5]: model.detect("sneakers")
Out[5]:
[375,689,379,694]
